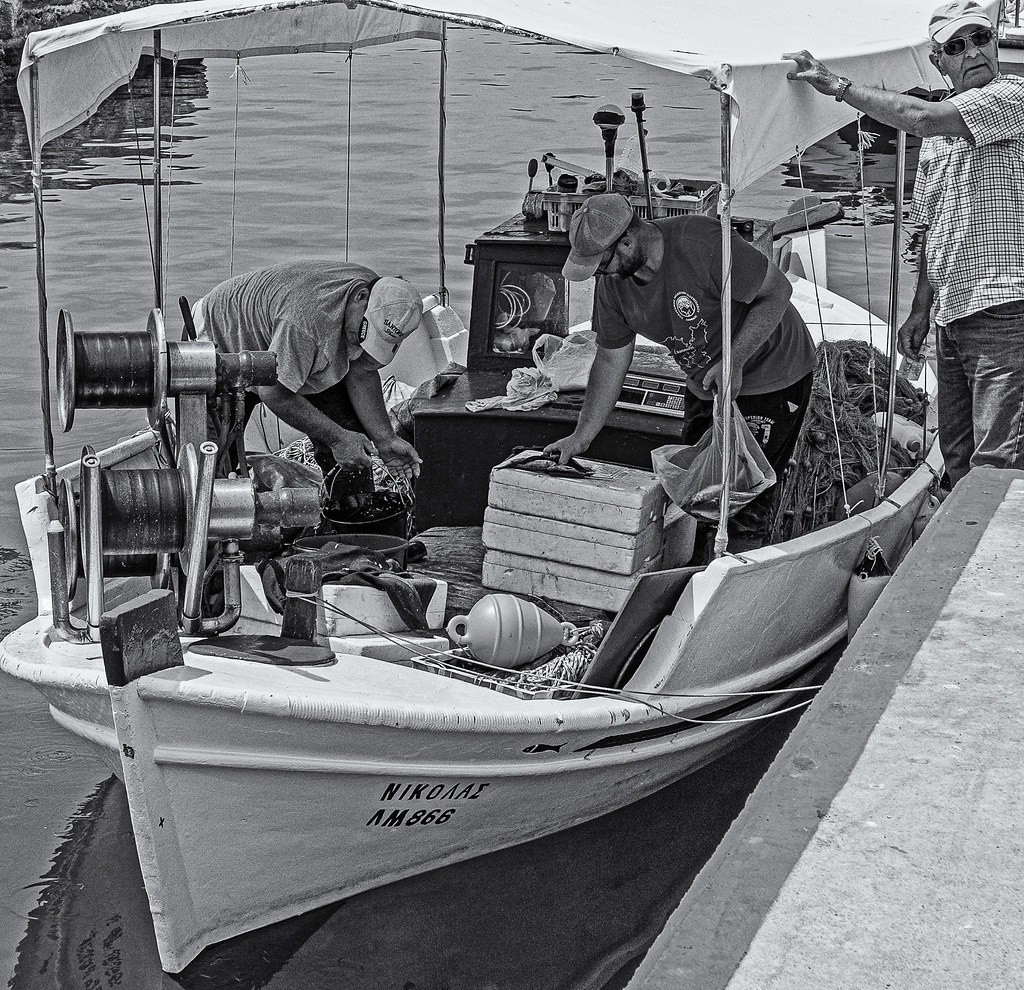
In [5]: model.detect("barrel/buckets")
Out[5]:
[320,457,414,571]
[292,534,409,572]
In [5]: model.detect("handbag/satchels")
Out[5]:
[651,392,776,522]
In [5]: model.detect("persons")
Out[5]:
[781,0,1024,492]
[179,260,422,627]
[543,192,818,560]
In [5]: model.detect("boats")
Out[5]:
[1,1,945,974]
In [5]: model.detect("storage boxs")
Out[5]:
[488,447,664,534]
[321,577,447,636]
[481,505,664,574]
[664,500,696,568]
[327,629,451,667]
[629,177,722,219]
[543,191,588,233]
[481,548,662,614]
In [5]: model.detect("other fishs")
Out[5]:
[492,453,597,479]
[677,451,771,524]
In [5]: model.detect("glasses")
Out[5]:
[595,242,619,275]
[937,28,994,56]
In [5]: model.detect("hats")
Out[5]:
[560,194,633,280]
[929,0,992,43]
[360,277,424,365]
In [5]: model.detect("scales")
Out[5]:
[610,348,689,419]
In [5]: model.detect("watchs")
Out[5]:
[835,77,851,101]
